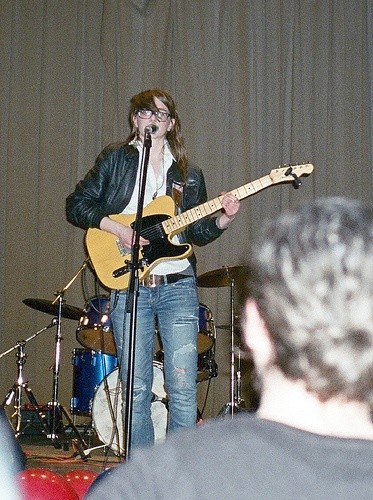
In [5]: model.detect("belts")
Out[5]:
[141,274,193,288]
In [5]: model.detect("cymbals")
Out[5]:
[197,266,252,288]
[21,298,83,320]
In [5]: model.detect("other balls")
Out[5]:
[15,469,79,500]
[63,471,100,500]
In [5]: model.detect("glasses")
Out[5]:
[138,109,171,122]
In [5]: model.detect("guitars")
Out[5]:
[85,158,315,290]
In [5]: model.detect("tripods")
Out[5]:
[14,262,86,462]
[0,318,59,438]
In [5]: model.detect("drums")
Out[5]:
[91,360,168,457]
[197,304,215,356]
[160,351,218,382]
[70,348,119,416]
[76,294,118,357]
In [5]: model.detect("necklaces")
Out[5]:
[152,172,164,200]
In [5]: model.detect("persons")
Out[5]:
[64,89,240,461]
[83,196,373,500]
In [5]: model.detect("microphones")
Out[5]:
[145,122,160,134]
